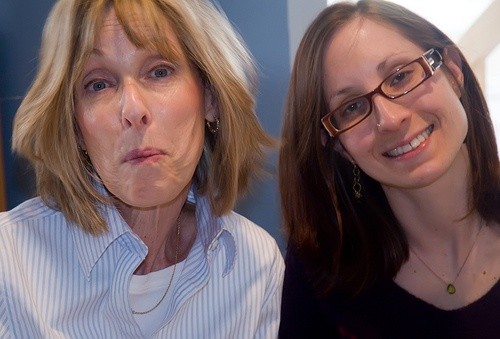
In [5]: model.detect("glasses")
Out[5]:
[321,46,444,138]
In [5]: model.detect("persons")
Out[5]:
[278,0,500,339]
[0,0,286,339]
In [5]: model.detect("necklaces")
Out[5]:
[407,214,484,294]
[130,211,183,314]
